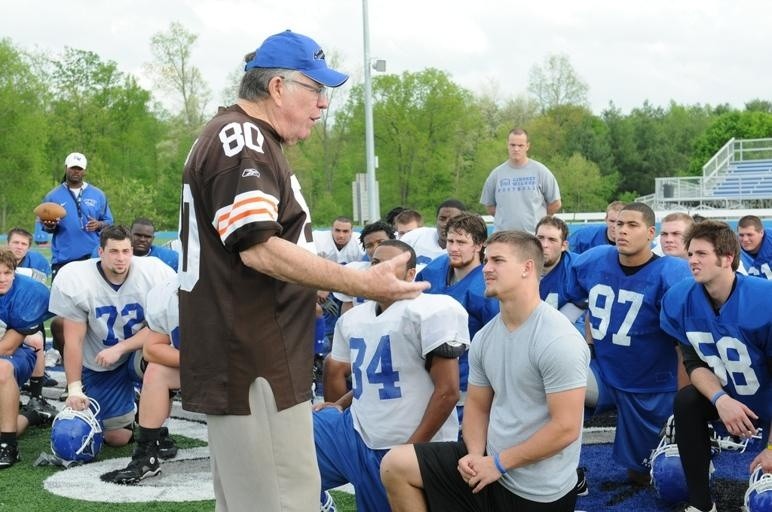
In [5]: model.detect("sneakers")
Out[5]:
[24,397,57,428]
[158,434,180,456]
[112,448,162,486]
[42,371,59,389]
[0,440,21,468]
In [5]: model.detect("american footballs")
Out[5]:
[32,202,67,221]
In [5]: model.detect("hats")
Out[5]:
[243,31,350,88]
[64,152,87,169]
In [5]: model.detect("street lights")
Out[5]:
[359,0,388,219]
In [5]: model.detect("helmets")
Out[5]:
[50,395,104,468]
[743,462,772,512]
[649,432,710,503]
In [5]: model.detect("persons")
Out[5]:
[480,127,563,239]
[180,29,431,512]
[40,153,114,286]
[0,217,182,487]
[312,199,772,512]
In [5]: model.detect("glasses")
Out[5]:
[276,74,327,95]
